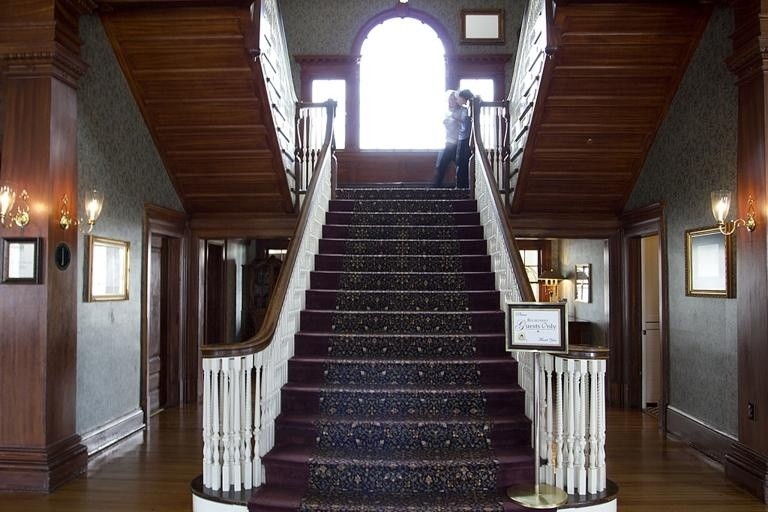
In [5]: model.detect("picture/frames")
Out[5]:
[0,235,42,286]
[458,8,505,43]
[683,225,734,300]
[572,264,591,303]
[83,233,131,303]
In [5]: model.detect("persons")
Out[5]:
[430,89,460,187]
[452,89,475,189]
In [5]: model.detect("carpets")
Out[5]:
[298,186,504,512]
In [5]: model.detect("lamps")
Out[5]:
[709,187,756,237]
[0,178,31,230]
[59,188,104,238]
